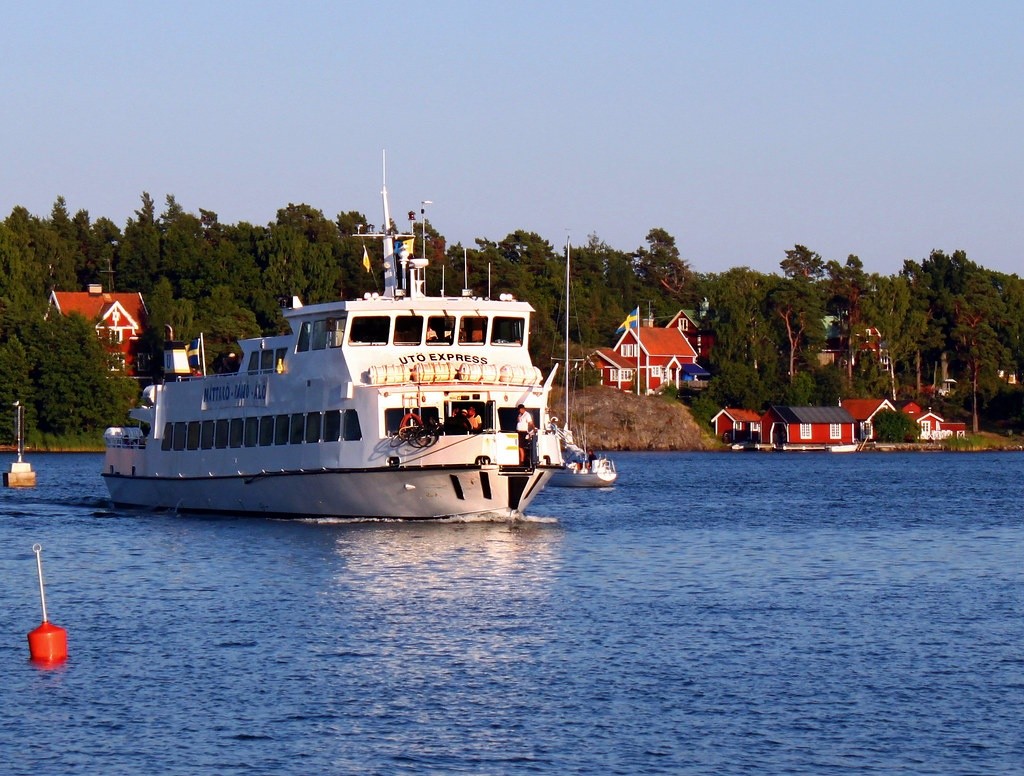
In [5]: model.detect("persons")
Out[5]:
[587,449,598,469]
[426,323,438,340]
[516,404,537,466]
[545,418,558,434]
[455,409,472,435]
[465,406,483,435]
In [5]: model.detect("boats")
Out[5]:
[102,145,566,522]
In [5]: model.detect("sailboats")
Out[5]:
[531,233,617,488]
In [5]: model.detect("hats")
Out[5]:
[518,404,524,409]
[462,409,468,413]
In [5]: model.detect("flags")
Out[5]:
[402,239,414,254]
[188,338,201,368]
[362,250,371,272]
[615,308,638,334]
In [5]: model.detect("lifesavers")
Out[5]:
[399,414,425,435]
[178,367,203,380]
